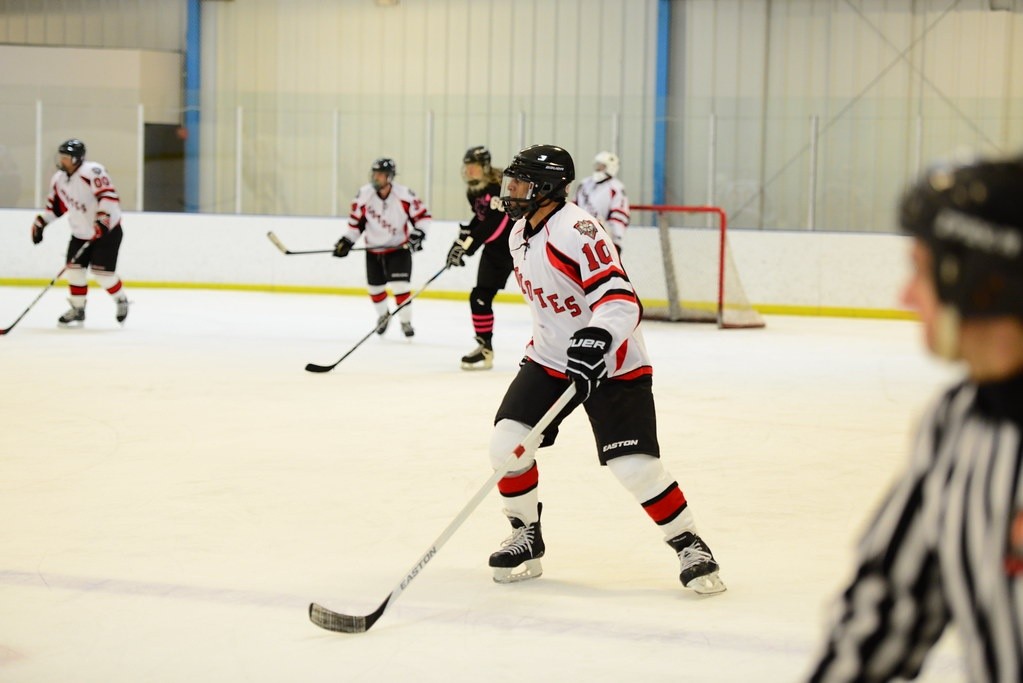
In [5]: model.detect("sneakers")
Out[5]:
[116,298,129,322]
[666,530,727,595]
[489,501,545,583]
[401,322,415,339]
[377,310,390,335]
[462,334,494,370]
[59,304,86,327]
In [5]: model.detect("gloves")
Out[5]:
[332,236,353,257]
[565,327,613,403]
[446,225,475,268]
[95,212,111,239]
[408,228,425,252]
[32,216,46,244]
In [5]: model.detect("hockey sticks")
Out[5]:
[305,258,458,373]
[306,383,578,634]
[0,232,99,337]
[265,230,404,255]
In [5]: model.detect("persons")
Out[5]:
[33,139,128,329]
[805,156,1023,683]
[446,146,523,370]
[576,152,630,259]
[333,159,432,337]
[490,145,726,596]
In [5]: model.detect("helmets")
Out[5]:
[593,151,619,184]
[500,145,575,222]
[463,147,491,185]
[371,159,395,190]
[893,160,1023,317]
[59,140,85,159]
